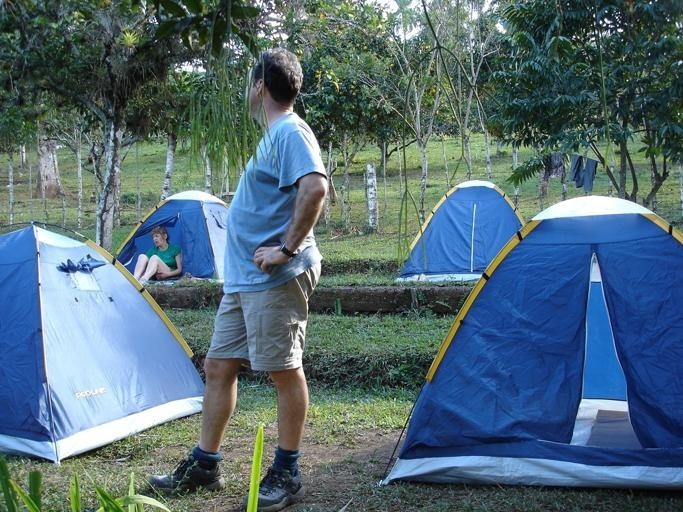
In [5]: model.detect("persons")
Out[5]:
[130,225,186,284]
[146,46,329,512]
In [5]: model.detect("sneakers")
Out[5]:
[143,454,225,494]
[138,278,150,287]
[241,467,304,512]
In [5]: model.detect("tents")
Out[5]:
[391,179,527,284]
[112,189,231,288]
[0,214,209,465]
[378,193,683,493]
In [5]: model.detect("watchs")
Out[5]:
[278,241,296,258]
